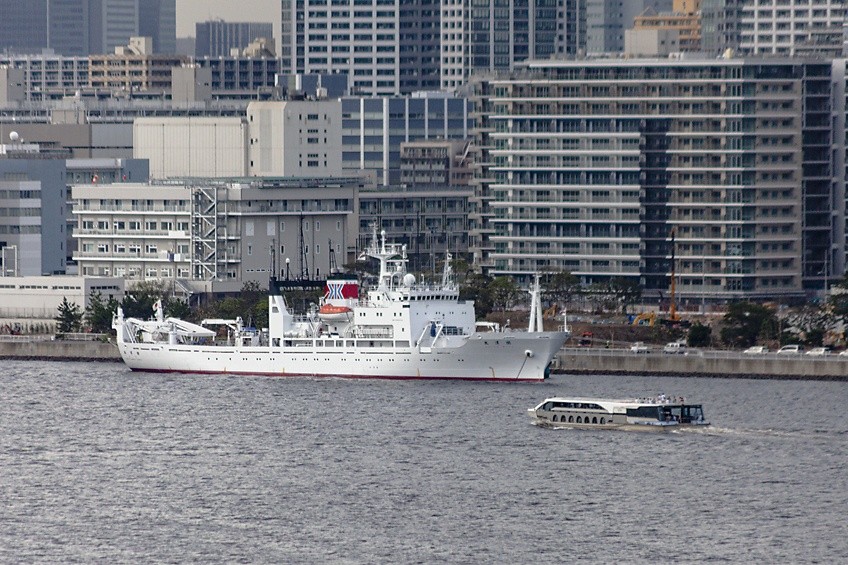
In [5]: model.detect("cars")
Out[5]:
[839,348,848,358]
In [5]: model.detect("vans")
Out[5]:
[663,343,686,354]
[778,344,805,354]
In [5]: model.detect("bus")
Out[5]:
[577,332,594,347]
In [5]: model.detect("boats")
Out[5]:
[317,304,353,322]
[111,218,573,382]
[527,394,710,428]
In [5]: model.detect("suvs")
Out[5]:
[805,348,831,357]
[744,346,768,355]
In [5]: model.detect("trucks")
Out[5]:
[630,342,654,353]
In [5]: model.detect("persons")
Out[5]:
[431,322,437,337]
[320,327,323,335]
[635,392,687,404]
[663,414,703,422]
[605,340,610,349]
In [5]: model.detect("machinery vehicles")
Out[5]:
[631,228,713,342]
